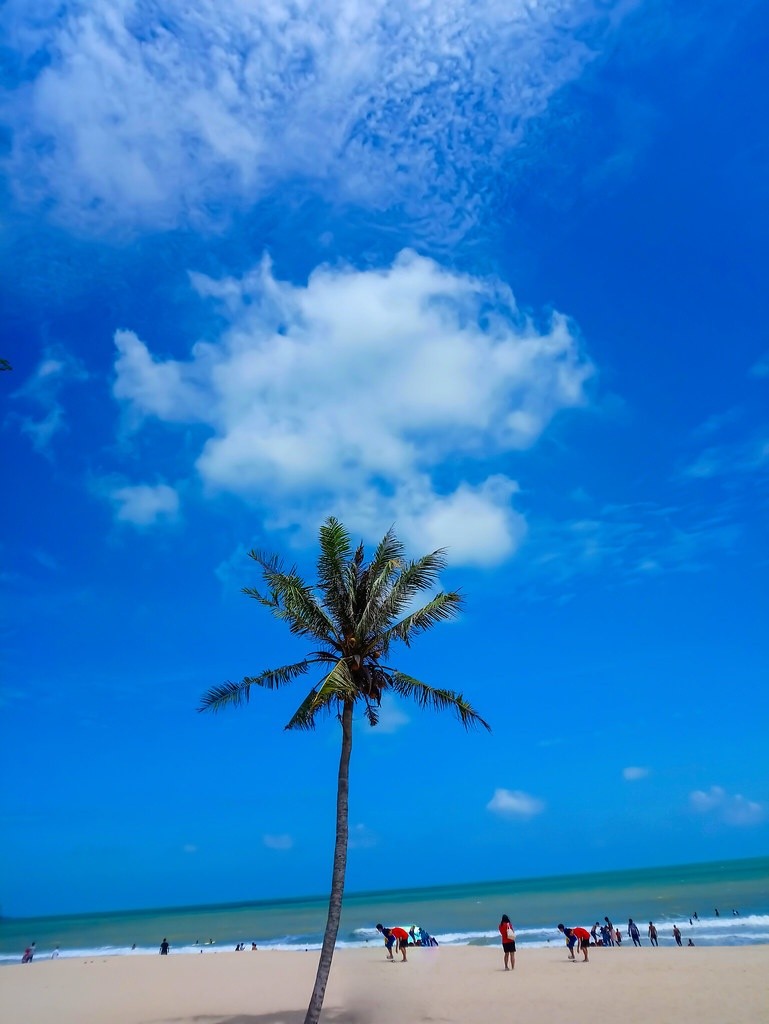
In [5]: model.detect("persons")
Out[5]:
[21,935,258,963]
[376,923,439,962]
[554,907,742,963]
[498,913,518,971]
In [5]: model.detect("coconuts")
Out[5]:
[349,638,356,648]
[351,662,360,670]
[373,652,380,658]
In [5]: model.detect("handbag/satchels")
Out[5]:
[507,923,515,941]
